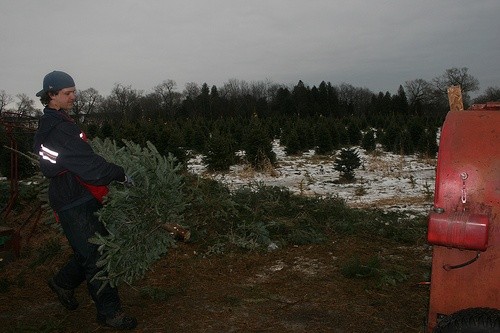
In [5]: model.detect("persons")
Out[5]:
[35,70,138,330]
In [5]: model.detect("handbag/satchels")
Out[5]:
[77,130,109,203]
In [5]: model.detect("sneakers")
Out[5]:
[95,310,136,331]
[48,277,79,311]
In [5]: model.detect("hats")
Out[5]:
[36,71,75,96]
